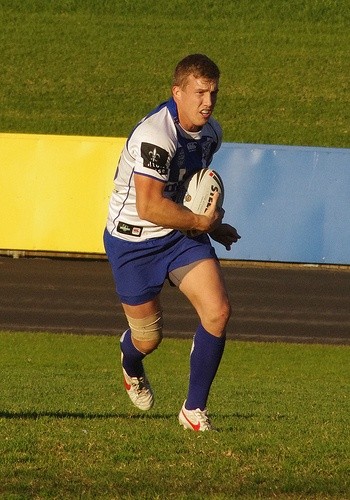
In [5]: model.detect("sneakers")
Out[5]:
[120,352,154,411]
[177,399,212,433]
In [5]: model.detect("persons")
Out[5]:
[103,53,242,432]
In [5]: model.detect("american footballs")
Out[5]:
[174,167,224,238]
